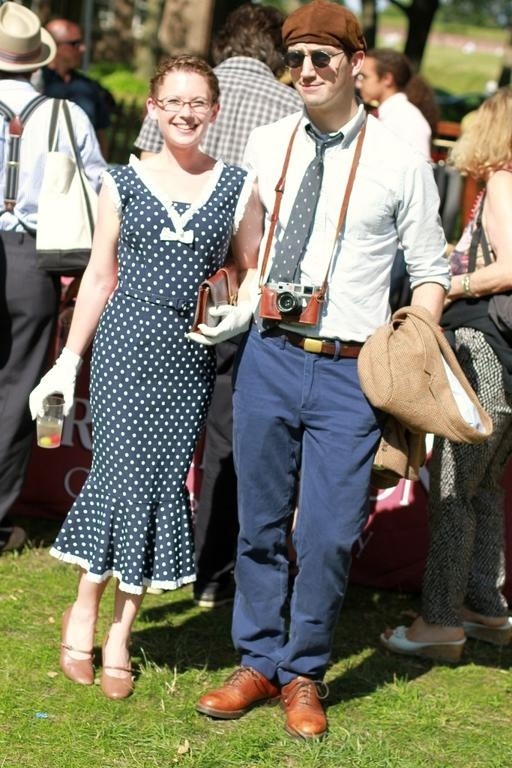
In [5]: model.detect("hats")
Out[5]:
[1,0,59,73]
[279,0,369,57]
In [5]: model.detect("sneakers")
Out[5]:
[1,523,29,552]
[195,587,236,609]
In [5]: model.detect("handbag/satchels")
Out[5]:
[487,293,511,335]
[34,100,100,275]
[192,266,238,335]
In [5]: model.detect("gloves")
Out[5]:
[29,347,85,422]
[186,303,251,346]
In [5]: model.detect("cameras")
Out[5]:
[267,281,319,316]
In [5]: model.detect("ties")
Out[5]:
[272,123,342,286]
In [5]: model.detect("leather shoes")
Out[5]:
[195,667,280,722]
[100,635,135,701]
[280,676,328,741]
[59,607,96,687]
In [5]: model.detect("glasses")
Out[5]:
[283,50,340,69]
[153,95,214,114]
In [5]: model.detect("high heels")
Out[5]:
[379,623,467,664]
[455,609,512,645]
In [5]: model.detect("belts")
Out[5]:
[272,329,361,362]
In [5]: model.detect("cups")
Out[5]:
[36,395,66,448]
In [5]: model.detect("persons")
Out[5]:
[1,0,511,651]
[190,2,454,738]
[26,52,264,701]
